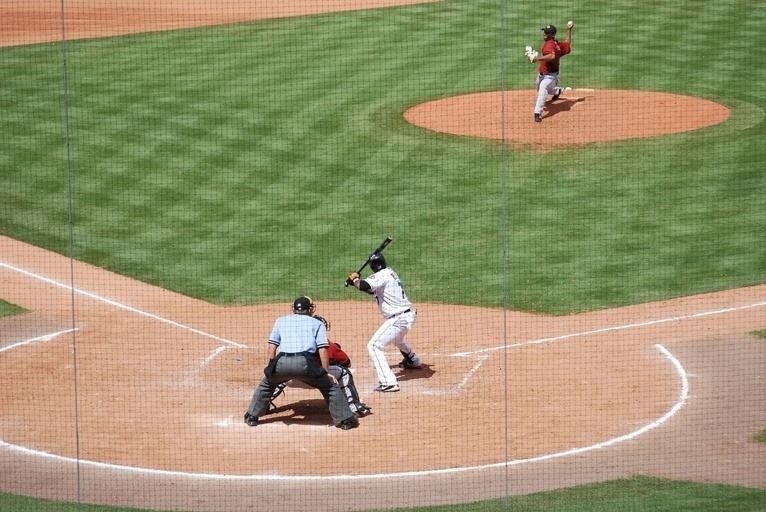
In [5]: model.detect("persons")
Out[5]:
[240,294,361,433]
[346,251,423,395]
[522,21,575,123]
[259,314,372,418]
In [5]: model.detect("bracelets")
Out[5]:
[352,276,360,284]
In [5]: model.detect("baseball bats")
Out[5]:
[344,236,392,287]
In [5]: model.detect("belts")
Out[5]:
[393,308,410,317]
[282,352,307,356]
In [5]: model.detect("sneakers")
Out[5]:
[399,358,422,369]
[244,412,257,426]
[534,114,540,122]
[341,403,372,430]
[374,381,400,392]
[552,89,562,101]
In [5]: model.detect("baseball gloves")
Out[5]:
[524,45,538,63]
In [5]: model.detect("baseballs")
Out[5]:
[567,20,573,25]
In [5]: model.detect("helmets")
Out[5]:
[369,253,386,273]
[540,25,556,35]
[293,295,313,314]
